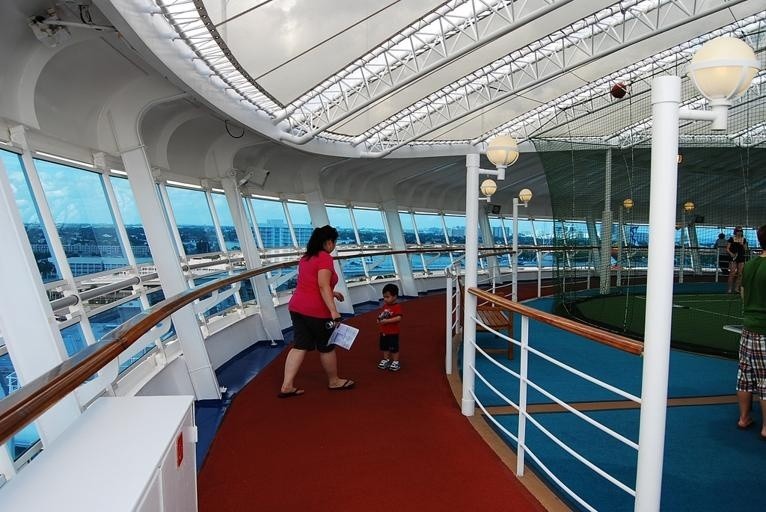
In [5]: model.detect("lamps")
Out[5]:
[240,167,269,187]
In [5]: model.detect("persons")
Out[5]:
[726,225,748,293]
[377,284,404,372]
[735,224,766,437]
[279,224,355,399]
[714,233,749,274]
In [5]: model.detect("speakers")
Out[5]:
[488,204,501,214]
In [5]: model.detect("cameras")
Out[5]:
[326,319,341,329]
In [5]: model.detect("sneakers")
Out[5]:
[377,359,401,371]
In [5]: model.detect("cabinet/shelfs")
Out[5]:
[0,394,200,512]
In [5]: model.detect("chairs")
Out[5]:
[458,275,513,360]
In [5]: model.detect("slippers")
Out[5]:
[330,378,354,390]
[279,379,303,396]
